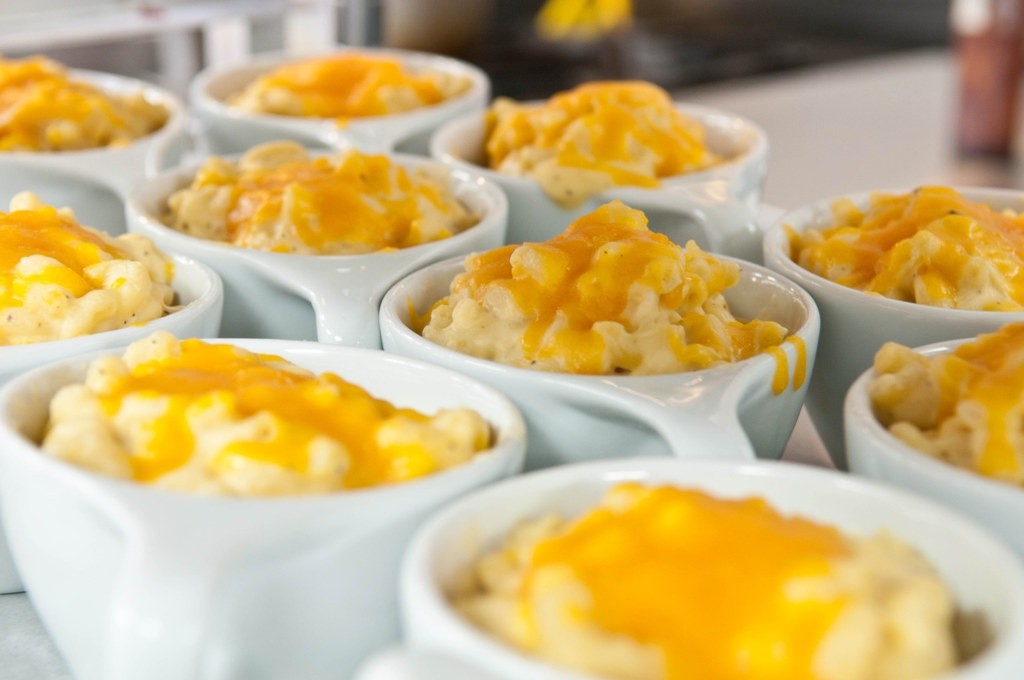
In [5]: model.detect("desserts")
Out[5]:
[2,42,1024,680]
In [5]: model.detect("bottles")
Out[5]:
[942,0,1024,189]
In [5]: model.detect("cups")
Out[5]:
[1,44,1023,680]
